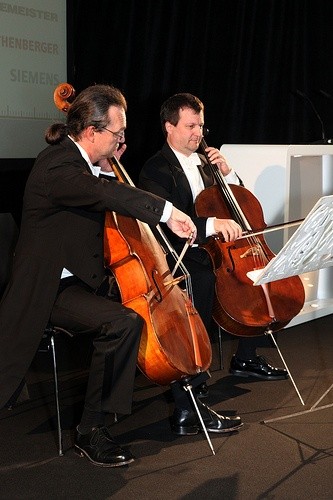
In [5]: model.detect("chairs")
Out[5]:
[0,213,119,457]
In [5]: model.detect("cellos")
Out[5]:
[192,128,306,407]
[52,83,217,457]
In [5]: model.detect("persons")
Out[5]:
[142,92,290,397]
[1,86,244,467]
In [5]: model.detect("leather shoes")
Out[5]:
[228,353,289,381]
[72,424,134,467]
[172,402,244,435]
[193,381,210,398]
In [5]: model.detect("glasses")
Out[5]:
[103,126,124,140]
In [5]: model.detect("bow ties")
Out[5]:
[92,166,101,178]
[175,153,201,169]
